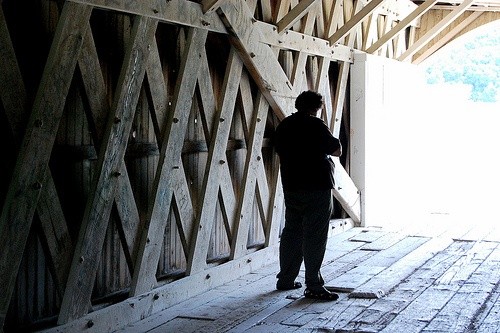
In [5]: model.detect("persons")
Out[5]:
[272,89,342,301]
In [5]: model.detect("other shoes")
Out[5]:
[276,280,302,291]
[304,287,339,301]
